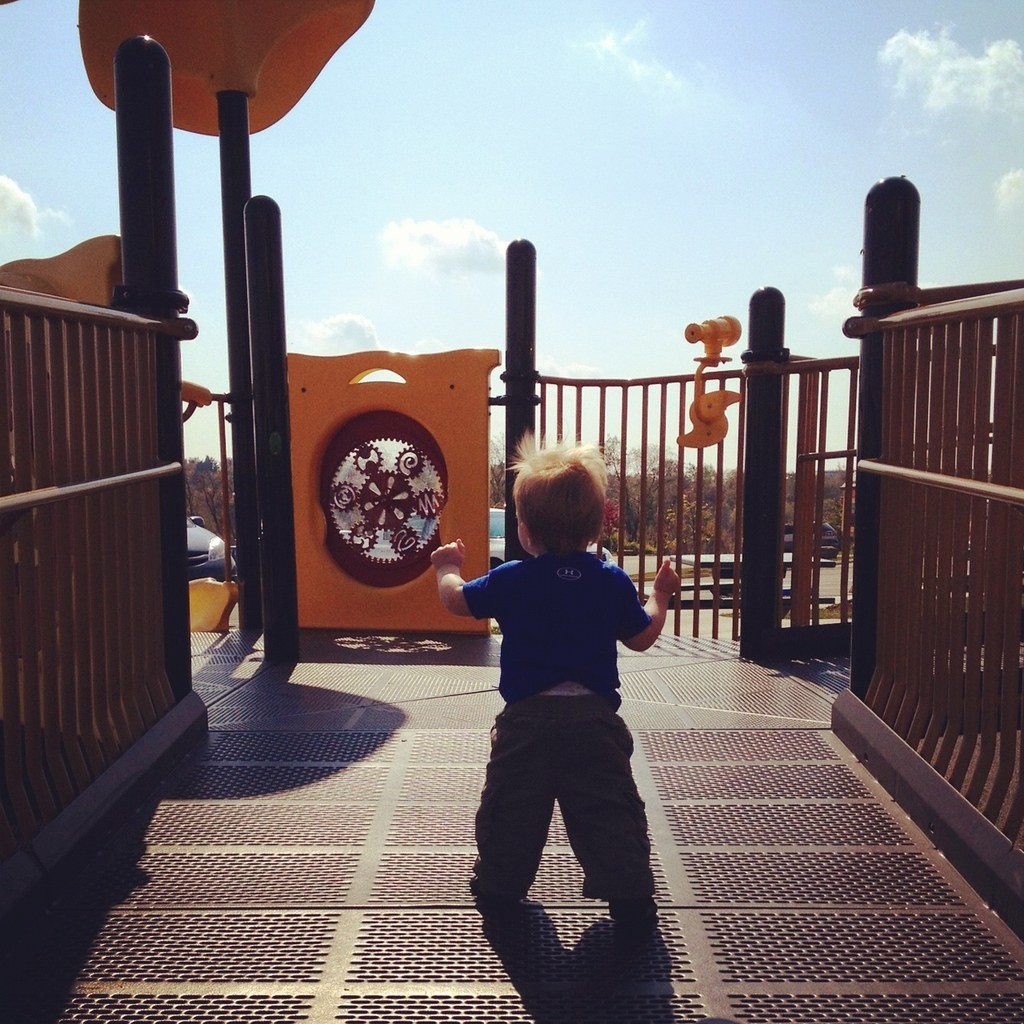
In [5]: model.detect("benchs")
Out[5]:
[660,585,839,608]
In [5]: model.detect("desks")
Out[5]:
[668,550,835,639]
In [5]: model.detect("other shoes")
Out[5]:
[471,876,528,906]
[610,899,658,923]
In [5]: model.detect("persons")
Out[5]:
[432,431,683,925]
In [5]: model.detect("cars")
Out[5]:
[785,518,840,559]
[184,515,238,584]
[489,508,616,571]
